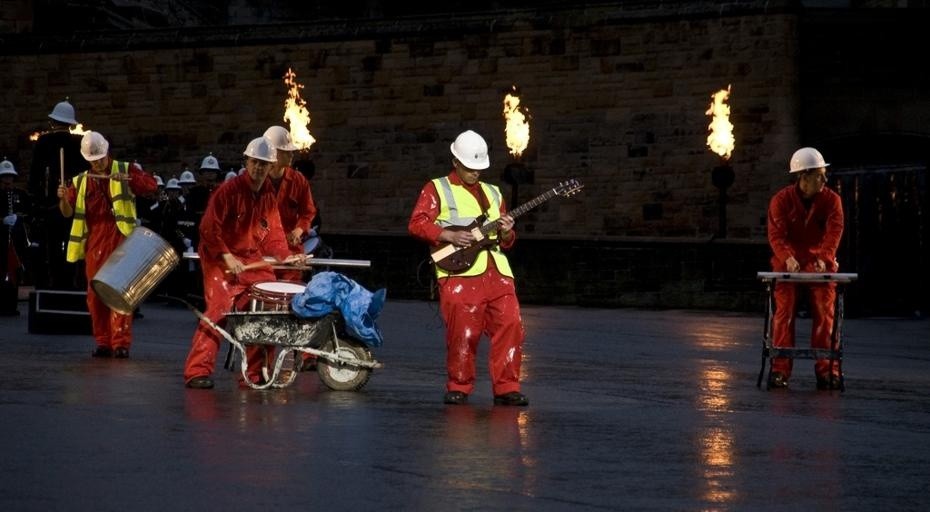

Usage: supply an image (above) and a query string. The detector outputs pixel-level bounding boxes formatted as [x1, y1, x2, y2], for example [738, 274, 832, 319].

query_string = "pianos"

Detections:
[757, 272, 859, 280]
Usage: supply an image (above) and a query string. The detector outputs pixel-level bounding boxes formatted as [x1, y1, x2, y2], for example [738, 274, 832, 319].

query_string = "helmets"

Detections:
[244, 137, 278, 162]
[225, 172, 235, 179]
[80, 132, 108, 161]
[451, 130, 489, 170]
[165, 179, 182, 189]
[177, 171, 196, 185]
[263, 126, 301, 151]
[200, 156, 221, 170]
[153, 176, 164, 186]
[0, 160, 17, 176]
[789, 147, 830, 173]
[48, 102, 77, 124]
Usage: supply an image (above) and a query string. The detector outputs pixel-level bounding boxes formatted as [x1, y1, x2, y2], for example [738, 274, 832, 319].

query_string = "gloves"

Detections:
[3, 216, 13, 226]
[11, 214, 18, 221]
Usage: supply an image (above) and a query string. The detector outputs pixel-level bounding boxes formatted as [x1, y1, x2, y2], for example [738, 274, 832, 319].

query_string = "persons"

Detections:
[185, 154, 222, 238]
[59, 132, 158, 359]
[185, 137, 309, 388]
[1, 157, 29, 317]
[29, 97, 91, 241]
[409, 130, 530, 406]
[151, 169, 197, 228]
[769, 148, 844, 391]
[264, 126, 319, 375]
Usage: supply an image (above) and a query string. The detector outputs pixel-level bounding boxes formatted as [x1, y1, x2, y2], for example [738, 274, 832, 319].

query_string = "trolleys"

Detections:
[193, 309, 385, 390]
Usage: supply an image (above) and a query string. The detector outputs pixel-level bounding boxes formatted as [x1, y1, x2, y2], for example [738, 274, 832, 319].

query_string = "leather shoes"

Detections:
[185, 377, 212, 388]
[768, 373, 789, 387]
[93, 348, 113, 356]
[494, 392, 529, 405]
[115, 348, 128, 358]
[817, 375, 845, 389]
[444, 391, 469, 403]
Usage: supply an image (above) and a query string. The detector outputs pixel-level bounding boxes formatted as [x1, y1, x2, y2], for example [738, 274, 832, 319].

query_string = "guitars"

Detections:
[430, 179, 585, 275]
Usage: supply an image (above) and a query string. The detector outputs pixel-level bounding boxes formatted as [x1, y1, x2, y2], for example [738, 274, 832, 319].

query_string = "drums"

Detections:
[250, 280, 307, 311]
[91, 226, 180, 316]
